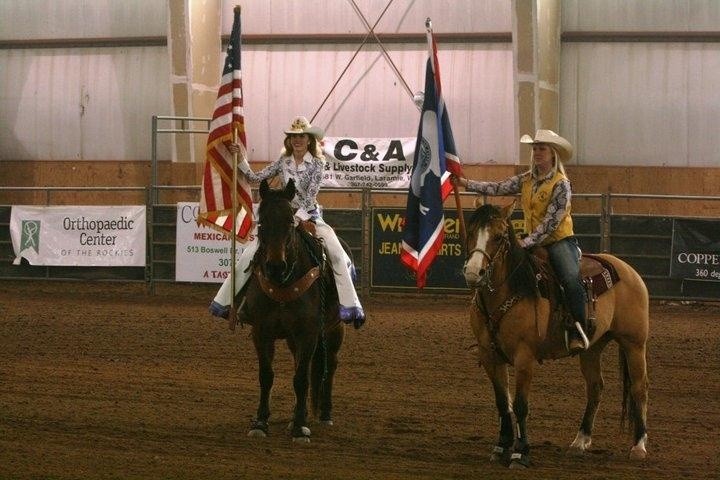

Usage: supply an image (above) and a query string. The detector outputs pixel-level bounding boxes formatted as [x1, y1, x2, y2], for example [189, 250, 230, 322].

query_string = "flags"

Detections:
[197, 6, 252, 245]
[399, 26, 461, 290]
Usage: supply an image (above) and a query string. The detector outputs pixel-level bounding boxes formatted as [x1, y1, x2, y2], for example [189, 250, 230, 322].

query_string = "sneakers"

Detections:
[570, 337, 585, 351]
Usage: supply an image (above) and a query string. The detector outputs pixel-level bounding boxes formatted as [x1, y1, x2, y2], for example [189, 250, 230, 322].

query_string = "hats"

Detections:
[519, 130, 574, 164]
[284, 116, 325, 142]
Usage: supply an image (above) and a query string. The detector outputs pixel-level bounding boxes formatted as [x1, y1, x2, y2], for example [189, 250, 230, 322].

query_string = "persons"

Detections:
[211, 115, 366, 329]
[452, 129, 588, 353]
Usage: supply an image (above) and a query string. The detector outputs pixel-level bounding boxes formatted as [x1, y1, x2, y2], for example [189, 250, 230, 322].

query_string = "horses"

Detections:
[460, 198, 652, 472]
[243, 176, 345, 444]
